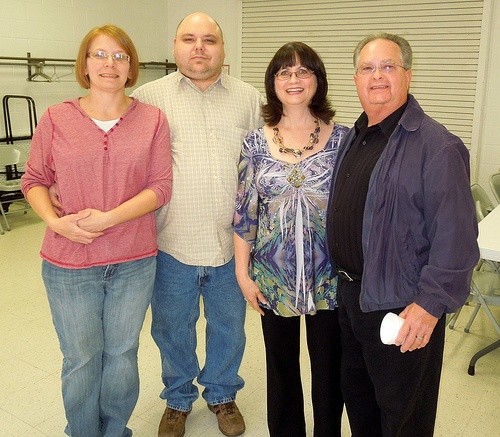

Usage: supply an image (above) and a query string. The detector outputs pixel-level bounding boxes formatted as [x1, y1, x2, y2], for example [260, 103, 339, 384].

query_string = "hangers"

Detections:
[27, 62, 84, 82]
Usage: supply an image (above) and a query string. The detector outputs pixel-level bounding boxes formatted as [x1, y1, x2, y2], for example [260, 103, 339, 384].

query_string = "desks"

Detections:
[468, 204, 500, 381]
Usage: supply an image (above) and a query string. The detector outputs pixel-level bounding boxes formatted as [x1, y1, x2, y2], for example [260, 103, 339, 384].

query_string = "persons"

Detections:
[50, 12, 268, 437]
[326, 33, 481, 437]
[20, 25, 172, 437]
[233, 41, 350, 437]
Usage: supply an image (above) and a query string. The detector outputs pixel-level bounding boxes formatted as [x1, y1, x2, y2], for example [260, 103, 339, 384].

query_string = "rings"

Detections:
[416, 335, 424, 340]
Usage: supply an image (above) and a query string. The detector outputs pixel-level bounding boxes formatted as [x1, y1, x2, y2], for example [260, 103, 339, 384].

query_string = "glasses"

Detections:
[273, 66, 315, 80]
[86, 50, 130, 64]
[356, 60, 407, 77]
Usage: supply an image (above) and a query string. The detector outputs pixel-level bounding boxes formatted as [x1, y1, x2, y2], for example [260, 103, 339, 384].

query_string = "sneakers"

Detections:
[158, 406, 191, 437]
[207, 401, 246, 437]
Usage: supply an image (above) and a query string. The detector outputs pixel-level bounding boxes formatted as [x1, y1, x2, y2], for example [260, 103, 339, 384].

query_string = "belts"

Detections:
[335, 268, 362, 282]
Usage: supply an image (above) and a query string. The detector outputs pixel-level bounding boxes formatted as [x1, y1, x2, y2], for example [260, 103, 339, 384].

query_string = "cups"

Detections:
[380, 312, 405, 345]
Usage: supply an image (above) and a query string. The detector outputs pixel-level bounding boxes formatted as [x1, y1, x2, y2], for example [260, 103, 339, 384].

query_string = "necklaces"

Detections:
[272, 115, 321, 159]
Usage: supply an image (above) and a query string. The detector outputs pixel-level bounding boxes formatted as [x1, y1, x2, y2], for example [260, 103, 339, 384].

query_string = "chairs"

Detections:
[0, 144, 28, 231]
[446, 170, 500, 332]
